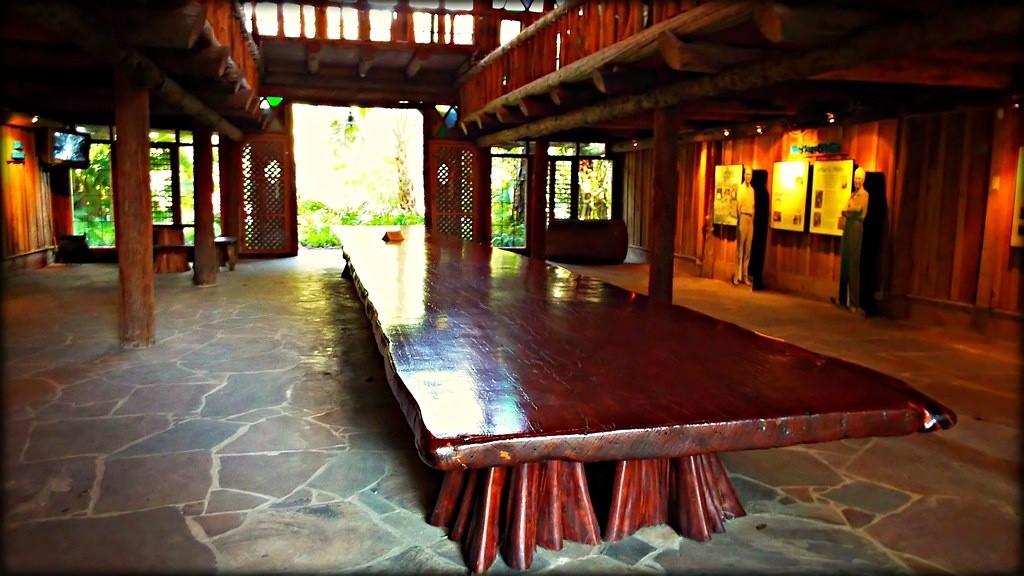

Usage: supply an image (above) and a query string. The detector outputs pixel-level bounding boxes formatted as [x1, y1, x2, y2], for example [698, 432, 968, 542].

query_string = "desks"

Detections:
[152, 223, 195, 274]
[330, 223, 926, 576]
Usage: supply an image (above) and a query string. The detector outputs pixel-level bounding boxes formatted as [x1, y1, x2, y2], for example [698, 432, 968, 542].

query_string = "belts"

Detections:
[741, 213, 752, 217]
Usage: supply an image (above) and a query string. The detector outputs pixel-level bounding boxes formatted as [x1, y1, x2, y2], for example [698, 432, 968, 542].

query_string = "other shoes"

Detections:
[733, 275, 738, 285]
[742, 274, 751, 285]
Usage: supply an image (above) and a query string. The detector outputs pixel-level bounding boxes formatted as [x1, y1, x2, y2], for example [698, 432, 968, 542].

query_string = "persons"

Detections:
[836, 166, 868, 312]
[733, 166, 755, 285]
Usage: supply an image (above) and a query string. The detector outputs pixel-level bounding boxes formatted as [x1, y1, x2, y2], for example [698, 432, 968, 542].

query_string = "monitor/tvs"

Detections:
[38, 128, 91, 167]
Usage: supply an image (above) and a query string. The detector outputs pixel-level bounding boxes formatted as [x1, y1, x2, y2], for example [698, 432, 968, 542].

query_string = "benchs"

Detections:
[155, 235, 237, 272]
